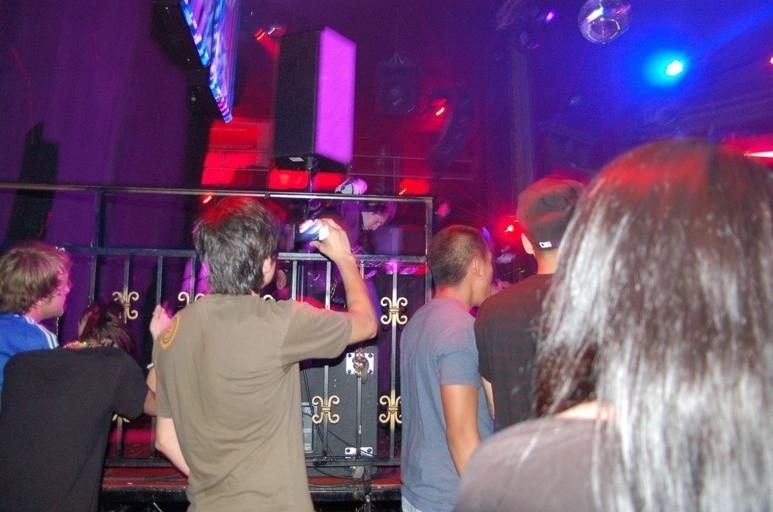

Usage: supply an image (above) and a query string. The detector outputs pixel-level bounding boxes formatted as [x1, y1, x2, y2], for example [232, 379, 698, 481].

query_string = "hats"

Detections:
[516, 180, 586, 254]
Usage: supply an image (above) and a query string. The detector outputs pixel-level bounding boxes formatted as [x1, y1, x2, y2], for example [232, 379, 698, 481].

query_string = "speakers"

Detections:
[274, 25, 356, 174]
[300, 274, 432, 474]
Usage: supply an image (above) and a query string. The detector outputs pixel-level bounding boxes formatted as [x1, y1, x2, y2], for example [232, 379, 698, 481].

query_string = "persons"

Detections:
[452, 136, 771, 511]
[470, 172, 587, 435]
[151, 198, 379, 511]
[0, 302, 172, 510]
[300, 174, 398, 438]
[0, 241, 71, 391]
[397, 223, 498, 512]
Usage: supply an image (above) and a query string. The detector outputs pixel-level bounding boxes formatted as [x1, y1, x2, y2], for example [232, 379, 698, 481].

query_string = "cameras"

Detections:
[295, 215, 330, 244]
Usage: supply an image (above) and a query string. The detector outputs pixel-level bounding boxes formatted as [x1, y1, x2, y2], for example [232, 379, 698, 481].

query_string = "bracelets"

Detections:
[146, 362, 154, 370]
